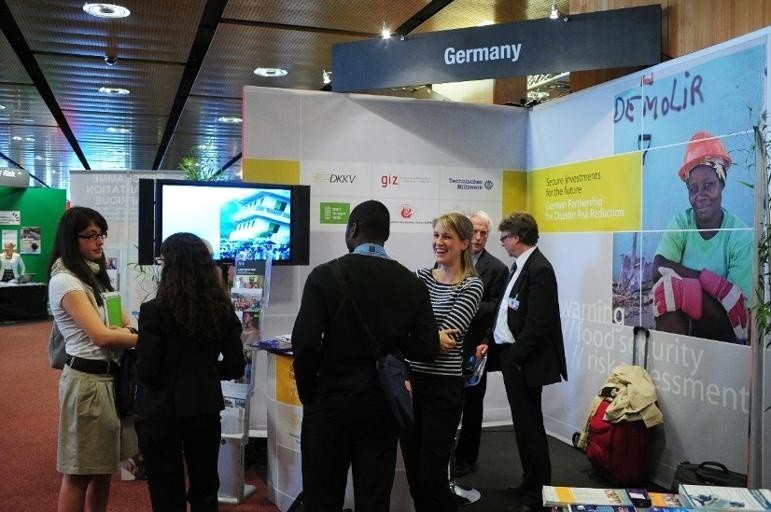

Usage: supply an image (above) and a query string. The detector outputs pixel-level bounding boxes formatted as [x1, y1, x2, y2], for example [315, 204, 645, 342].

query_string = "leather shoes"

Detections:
[451, 459, 480, 478]
[506, 481, 552, 512]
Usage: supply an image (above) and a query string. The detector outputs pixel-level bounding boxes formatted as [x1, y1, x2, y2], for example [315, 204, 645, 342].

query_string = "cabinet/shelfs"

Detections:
[0, 280, 48, 324]
[217, 250, 273, 505]
[264, 349, 410, 511]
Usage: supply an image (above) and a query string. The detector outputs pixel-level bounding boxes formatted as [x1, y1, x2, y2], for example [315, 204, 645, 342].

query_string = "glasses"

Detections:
[500, 233, 511, 242]
[78, 231, 107, 241]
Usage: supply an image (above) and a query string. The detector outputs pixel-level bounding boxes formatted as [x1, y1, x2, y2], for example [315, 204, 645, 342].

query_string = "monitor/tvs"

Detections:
[136, 177, 310, 267]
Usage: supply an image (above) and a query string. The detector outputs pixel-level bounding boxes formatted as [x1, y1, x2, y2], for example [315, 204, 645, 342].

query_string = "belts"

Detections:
[64, 354, 117, 375]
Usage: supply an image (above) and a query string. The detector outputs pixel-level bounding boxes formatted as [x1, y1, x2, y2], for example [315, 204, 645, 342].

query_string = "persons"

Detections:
[398, 213, 485, 512]
[134, 232, 247, 512]
[291, 199, 440, 512]
[0, 241, 26, 282]
[650, 131, 754, 345]
[475, 212, 568, 512]
[450, 210, 510, 477]
[47, 207, 139, 512]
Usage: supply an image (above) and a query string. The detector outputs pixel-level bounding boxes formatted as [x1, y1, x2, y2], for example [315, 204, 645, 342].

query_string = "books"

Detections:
[214, 260, 266, 384]
[541, 483, 771, 512]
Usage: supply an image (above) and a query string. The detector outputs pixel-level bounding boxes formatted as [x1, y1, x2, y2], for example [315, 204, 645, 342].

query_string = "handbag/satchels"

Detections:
[376, 354, 414, 433]
[47, 319, 66, 370]
[114, 349, 138, 420]
[670, 461, 747, 493]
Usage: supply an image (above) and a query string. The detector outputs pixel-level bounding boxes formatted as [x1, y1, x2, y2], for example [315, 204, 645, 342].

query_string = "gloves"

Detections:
[648, 266, 704, 320]
[699, 268, 750, 339]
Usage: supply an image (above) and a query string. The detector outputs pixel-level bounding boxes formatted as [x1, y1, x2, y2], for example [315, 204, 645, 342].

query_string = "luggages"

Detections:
[586, 326, 650, 483]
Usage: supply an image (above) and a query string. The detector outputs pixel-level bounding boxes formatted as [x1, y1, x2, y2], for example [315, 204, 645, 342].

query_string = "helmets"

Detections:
[678, 131, 732, 183]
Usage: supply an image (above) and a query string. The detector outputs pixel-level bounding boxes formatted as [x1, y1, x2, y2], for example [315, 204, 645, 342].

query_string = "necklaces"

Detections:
[432, 269, 460, 305]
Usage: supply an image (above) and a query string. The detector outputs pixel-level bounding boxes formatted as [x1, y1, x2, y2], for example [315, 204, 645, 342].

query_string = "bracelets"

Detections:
[125, 326, 138, 334]
